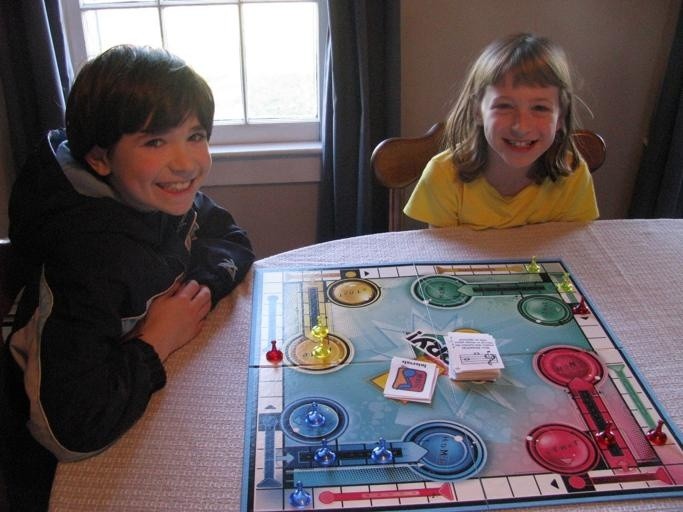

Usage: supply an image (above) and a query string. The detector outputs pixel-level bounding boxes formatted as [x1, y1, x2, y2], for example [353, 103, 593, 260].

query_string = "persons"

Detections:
[402, 32, 604, 231]
[0, 44, 258, 511]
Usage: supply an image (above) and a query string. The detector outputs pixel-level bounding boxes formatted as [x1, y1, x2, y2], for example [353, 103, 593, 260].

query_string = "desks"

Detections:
[46, 218, 681, 512]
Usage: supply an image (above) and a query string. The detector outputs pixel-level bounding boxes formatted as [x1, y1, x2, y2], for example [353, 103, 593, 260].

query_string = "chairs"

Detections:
[366, 117, 611, 234]
[0, 236, 26, 347]
